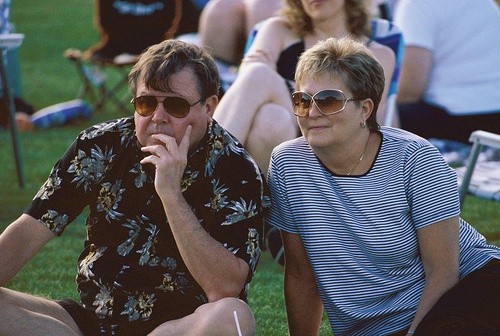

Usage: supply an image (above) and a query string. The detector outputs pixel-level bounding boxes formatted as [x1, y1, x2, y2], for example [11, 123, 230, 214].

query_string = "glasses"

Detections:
[130, 95, 201, 118]
[291, 88, 359, 116]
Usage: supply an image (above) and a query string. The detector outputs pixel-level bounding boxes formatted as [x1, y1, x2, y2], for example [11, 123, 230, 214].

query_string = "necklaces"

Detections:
[345, 128, 372, 177]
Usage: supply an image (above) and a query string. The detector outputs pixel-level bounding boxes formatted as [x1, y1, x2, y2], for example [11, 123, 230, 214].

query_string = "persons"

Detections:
[260, 37, 499, 335]
[378, 0, 500, 145]
[196, 0, 289, 96]
[211, 0, 396, 195]
[1, 37, 271, 336]
[84, 0, 199, 68]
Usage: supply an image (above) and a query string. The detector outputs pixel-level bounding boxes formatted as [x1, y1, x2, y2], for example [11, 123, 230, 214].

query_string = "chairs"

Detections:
[64, 1, 184, 117]
[460, 131, 500, 213]
[244, 15, 404, 126]
